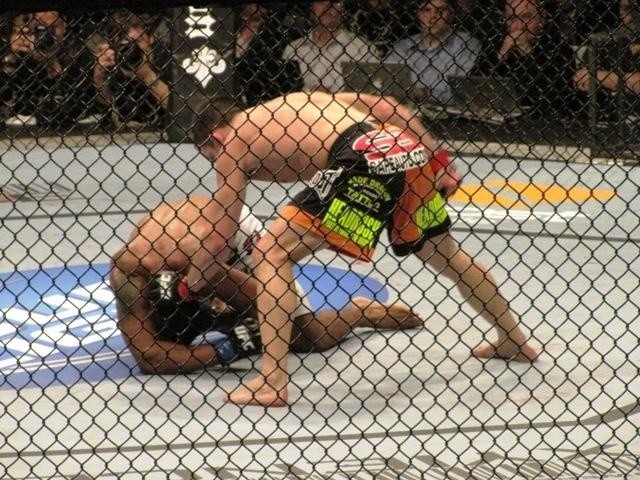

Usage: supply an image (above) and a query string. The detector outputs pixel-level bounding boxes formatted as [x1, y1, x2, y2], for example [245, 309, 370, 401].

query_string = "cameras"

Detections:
[115, 40, 144, 70]
[33, 25, 56, 51]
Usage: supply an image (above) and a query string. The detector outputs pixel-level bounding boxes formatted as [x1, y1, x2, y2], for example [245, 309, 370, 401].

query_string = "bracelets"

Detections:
[426, 148, 452, 179]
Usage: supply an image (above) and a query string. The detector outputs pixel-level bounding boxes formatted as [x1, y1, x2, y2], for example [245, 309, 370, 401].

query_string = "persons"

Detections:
[146, 86, 539, 408]
[1, 0, 639, 127]
[109, 192, 424, 377]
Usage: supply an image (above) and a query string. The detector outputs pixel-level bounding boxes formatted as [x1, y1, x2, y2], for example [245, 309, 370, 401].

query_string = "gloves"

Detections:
[149, 272, 194, 310]
[213, 317, 263, 365]
[429, 149, 461, 198]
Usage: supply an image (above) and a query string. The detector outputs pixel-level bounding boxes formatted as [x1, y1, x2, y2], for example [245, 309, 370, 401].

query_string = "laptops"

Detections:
[447, 75, 514, 110]
[235, 57, 306, 106]
[340, 63, 412, 104]
